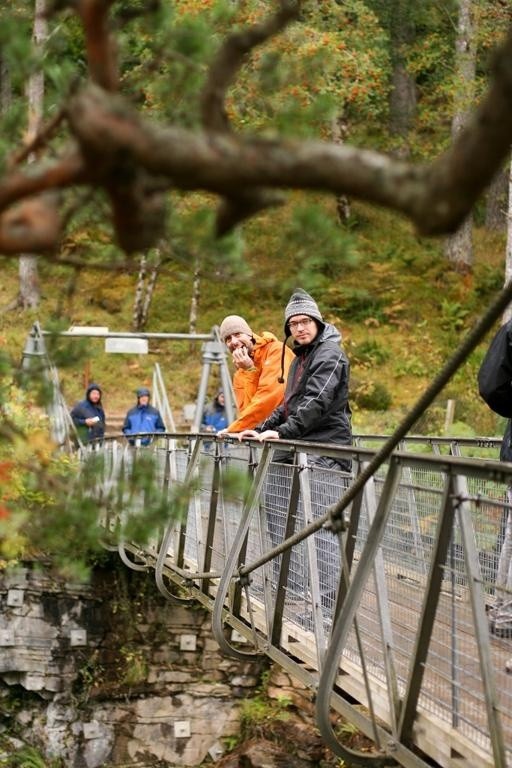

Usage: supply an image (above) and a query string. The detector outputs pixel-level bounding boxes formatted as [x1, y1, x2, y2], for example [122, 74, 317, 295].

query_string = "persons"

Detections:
[123, 388, 166, 446]
[69, 385, 105, 450]
[478, 312, 512, 638]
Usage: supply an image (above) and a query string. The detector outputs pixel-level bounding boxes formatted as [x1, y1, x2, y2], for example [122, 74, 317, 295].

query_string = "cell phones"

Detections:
[92, 416, 100, 422]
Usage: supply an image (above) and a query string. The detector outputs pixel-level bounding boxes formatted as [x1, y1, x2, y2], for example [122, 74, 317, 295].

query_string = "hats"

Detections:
[282, 288, 325, 338]
[219, 314, 253, 344]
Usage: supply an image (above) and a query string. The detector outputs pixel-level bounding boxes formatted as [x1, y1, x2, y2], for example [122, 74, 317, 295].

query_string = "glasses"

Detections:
[286, 317, 314, 329]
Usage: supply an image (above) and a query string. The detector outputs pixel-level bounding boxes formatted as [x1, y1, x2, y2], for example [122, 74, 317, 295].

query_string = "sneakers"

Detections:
[486, 619, 512, 638]
[252, 574, 333, 634]
